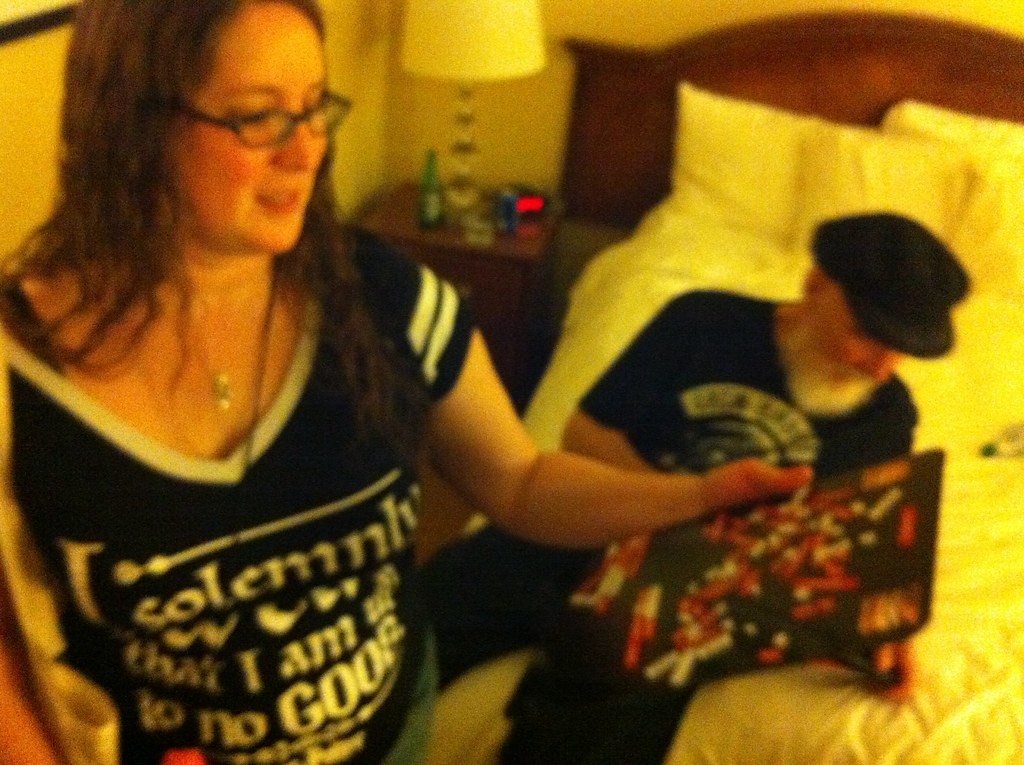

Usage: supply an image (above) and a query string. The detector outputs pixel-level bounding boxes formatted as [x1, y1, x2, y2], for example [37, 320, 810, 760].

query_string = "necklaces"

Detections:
[209, 366, 238, 416]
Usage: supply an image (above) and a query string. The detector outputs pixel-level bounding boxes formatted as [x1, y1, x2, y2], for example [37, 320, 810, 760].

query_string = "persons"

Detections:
[0, 0, 811, 764]
[415, 213, 968, 765]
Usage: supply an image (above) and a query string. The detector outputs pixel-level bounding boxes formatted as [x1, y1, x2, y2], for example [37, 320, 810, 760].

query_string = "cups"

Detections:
[462, 201, 496, 246]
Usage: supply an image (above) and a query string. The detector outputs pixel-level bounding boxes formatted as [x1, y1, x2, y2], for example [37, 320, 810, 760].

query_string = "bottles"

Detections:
[421, 150, 442, 230]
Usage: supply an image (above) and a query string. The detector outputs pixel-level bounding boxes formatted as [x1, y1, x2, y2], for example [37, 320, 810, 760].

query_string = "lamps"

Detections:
[398, 0, 548, 206]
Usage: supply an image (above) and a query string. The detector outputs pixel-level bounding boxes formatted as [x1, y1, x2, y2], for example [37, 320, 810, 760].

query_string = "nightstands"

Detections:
[353, 182, 565, 394]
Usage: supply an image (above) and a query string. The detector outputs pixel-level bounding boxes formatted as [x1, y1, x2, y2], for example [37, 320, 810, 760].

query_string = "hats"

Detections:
[810, 212, 973, 359]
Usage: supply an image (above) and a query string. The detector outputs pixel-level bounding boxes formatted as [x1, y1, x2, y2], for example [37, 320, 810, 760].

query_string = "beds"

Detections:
[414, 12, 1024, 765]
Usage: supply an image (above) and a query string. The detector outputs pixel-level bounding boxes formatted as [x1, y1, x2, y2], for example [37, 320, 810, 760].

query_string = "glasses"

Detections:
[171, 92, 353, 150]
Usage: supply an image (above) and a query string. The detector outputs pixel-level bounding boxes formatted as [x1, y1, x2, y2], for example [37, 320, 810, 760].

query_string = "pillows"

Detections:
[671, 83, 967, 253]
[876, 101, 1024, 303]
